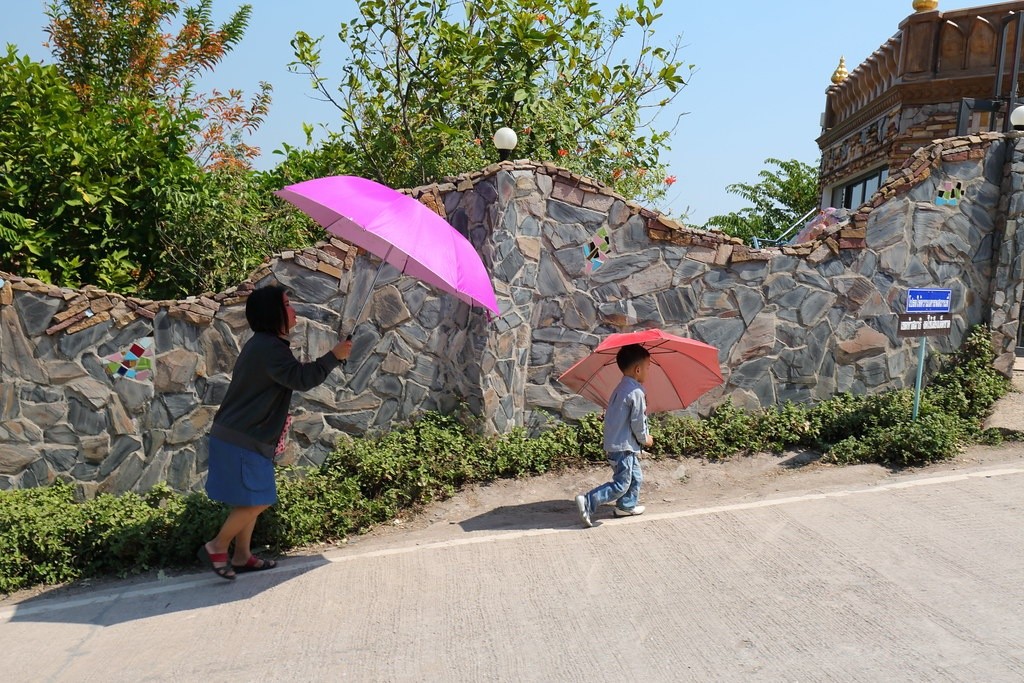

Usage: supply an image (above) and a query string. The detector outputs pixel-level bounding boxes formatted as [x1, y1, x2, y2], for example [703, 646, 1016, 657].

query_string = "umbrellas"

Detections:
[274, 176, 500, 366]
[556, 328, 725, 450]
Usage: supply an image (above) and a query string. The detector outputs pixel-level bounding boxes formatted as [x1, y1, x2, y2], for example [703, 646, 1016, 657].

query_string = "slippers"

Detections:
[197, 546, 237, 579]
[231, 556, 276, 573]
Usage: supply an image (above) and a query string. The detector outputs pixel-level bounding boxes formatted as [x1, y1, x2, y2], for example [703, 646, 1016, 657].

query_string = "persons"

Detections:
[204, 286, 353, 580]
[575, 344, 654, 527]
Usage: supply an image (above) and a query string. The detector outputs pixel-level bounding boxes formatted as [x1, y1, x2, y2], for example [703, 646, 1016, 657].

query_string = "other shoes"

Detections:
[613, 506, 645, 517]
[575, 495, 593, 526]
